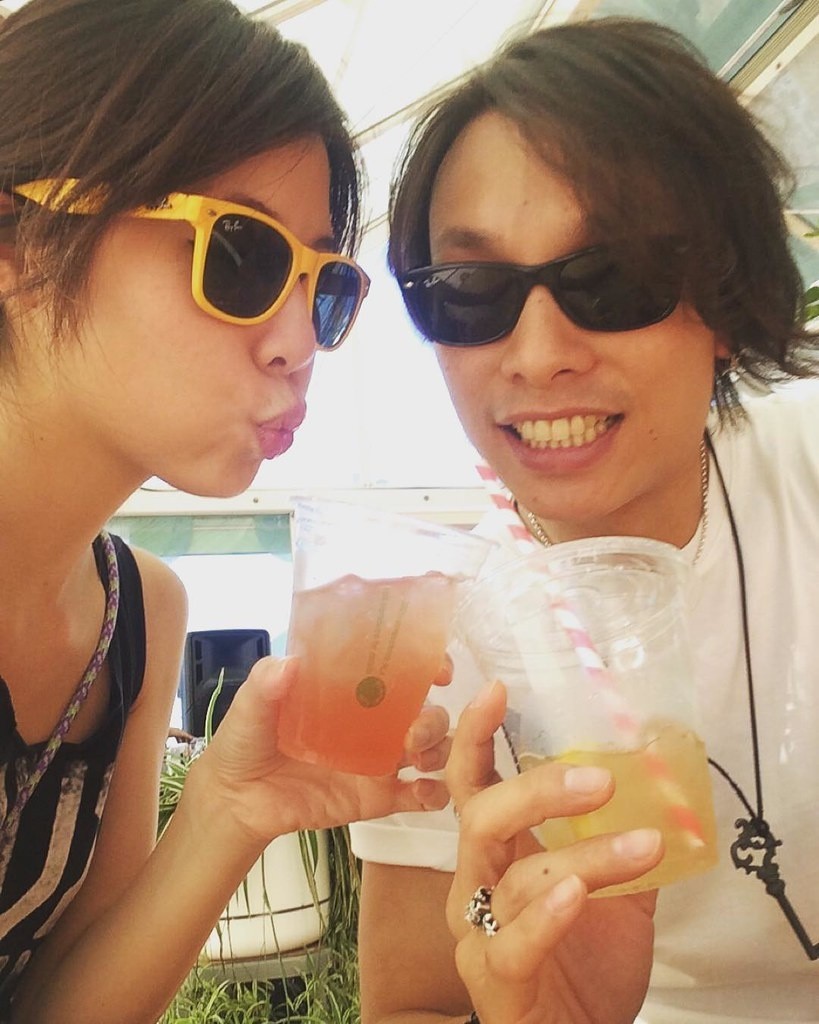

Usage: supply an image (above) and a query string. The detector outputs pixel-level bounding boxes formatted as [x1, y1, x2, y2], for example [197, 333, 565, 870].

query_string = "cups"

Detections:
[279, 492, 494, 778]
[451, 534, 720, 898]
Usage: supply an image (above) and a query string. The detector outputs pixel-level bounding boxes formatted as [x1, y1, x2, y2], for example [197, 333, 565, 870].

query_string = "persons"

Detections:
[0, 2, 453, 1024]
[356, 21, 819, 1021]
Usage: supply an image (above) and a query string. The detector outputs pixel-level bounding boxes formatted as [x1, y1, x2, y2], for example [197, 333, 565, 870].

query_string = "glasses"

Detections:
[11, 178, 371, 351]
[393, 232, 689, 348]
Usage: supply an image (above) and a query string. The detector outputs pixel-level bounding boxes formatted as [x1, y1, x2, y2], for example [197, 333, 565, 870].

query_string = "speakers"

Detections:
[176, 628, 271, 744]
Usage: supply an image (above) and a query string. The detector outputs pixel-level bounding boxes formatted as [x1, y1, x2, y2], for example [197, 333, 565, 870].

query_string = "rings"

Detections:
[466, 886, 501, 936]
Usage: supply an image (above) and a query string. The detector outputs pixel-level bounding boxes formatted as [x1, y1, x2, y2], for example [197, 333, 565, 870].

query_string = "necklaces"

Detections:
[527, 434, 711, 582]
[512, 425, 819, 963]
